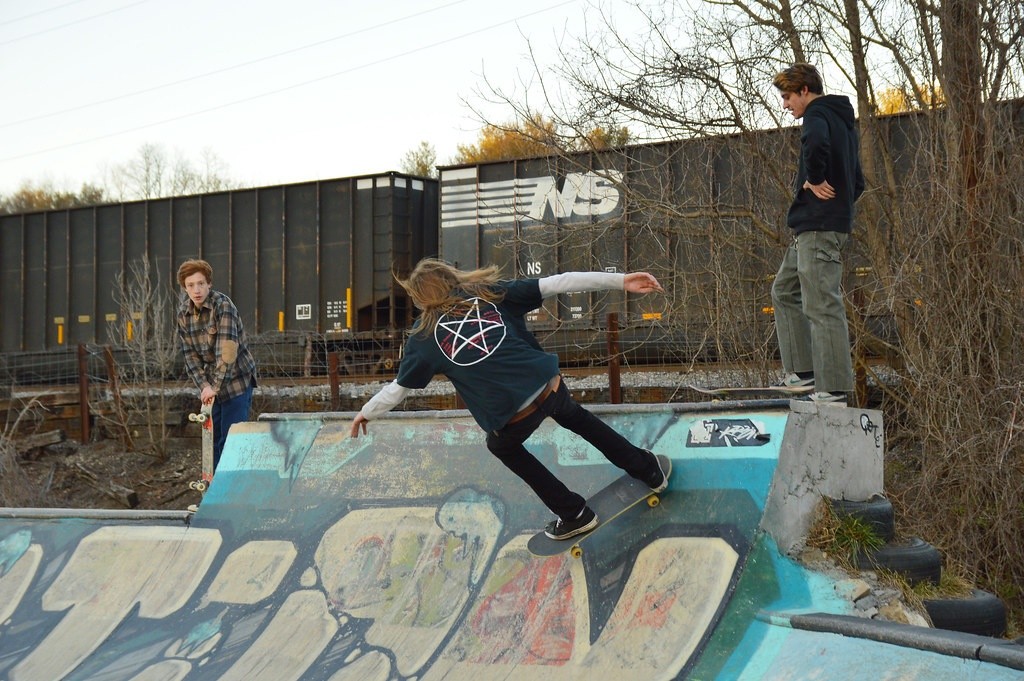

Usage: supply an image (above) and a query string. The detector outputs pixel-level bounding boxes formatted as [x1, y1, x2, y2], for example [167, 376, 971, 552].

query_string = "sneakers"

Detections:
[794, 389, 848, 406]
[544, 505, 599, 540]
[640, 447, 668, 493]
[770, 371, 815, 388]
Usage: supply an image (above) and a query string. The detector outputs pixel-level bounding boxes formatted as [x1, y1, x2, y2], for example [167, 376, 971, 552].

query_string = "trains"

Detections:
[1, 94, 1024, 386]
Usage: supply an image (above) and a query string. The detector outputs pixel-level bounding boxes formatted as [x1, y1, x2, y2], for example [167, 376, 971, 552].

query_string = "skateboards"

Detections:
[527, 453, 672, 558]
[188, 396, 215, 500]
[687, 383, 814, 401]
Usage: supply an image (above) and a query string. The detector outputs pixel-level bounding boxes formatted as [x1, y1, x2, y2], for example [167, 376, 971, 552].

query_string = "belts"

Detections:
[508, 384, 552, 424]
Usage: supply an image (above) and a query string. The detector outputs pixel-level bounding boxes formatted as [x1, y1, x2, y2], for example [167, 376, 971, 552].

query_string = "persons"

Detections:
[771, 63, 866, 406]
[349, 258, 668, 542]
[177, 259, 259, 476]
